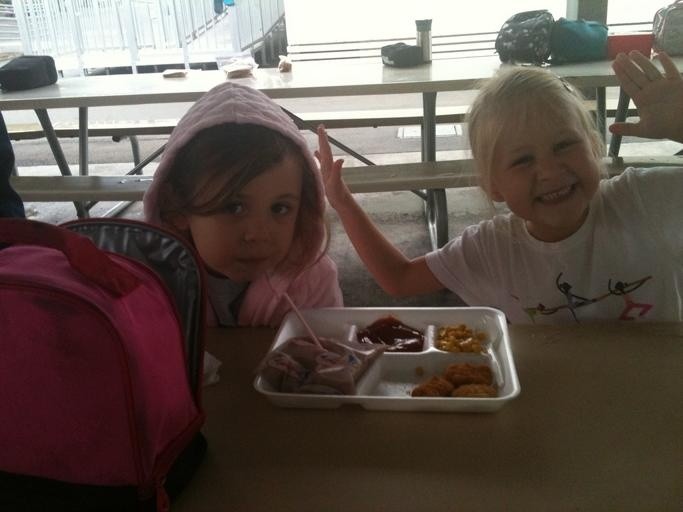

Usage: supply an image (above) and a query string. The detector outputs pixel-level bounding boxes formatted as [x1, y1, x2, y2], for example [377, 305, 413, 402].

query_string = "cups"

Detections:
[415, 19, 432, 64]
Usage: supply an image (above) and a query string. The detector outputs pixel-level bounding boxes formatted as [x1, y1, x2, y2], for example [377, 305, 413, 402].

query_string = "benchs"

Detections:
[6, 158, 683, 293]
[1, 92, 636, 176]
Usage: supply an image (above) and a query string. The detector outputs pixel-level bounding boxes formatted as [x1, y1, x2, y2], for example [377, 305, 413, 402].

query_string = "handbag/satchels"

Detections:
[652, 0, 683, 56]
[550, 17, 608, 65]
[1, 217, 205, 492]
[0, 56, 58, 91]
[495, 9, 554, 64]
[381, 43, 422, 68]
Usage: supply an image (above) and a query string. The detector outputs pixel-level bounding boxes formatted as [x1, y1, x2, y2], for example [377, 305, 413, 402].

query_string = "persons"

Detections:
[0, 112, 26, 221]
[309, 51, 683, 328]
[142, 83, 341, 334]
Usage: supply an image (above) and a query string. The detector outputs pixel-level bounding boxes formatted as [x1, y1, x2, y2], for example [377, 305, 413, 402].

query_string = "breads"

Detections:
[412, 369, 451, 398]
[441, 363, 492, 383]
[451, 382, 495, 401]
